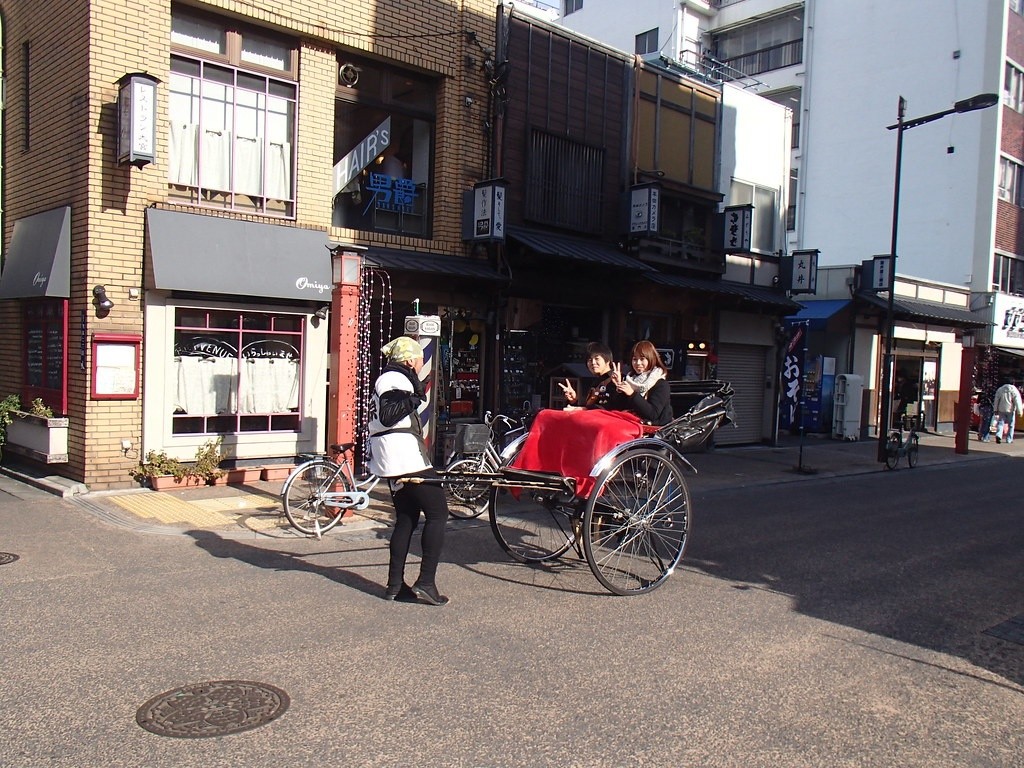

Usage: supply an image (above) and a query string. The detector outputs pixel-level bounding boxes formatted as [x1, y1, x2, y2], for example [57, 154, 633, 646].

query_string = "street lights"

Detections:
[876, 93, 999, 462]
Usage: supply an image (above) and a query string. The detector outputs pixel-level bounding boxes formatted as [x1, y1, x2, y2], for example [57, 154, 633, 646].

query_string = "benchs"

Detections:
[641, 392, 711, 433]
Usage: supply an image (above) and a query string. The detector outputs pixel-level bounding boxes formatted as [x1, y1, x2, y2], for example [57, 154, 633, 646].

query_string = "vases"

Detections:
[226, 465, 265, 483]
[262, 465, 310, 480]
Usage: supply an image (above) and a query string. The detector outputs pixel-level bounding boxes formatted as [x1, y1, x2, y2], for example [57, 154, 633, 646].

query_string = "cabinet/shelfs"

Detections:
[371, 175, 427, 237]
[550, 376, 596, 410]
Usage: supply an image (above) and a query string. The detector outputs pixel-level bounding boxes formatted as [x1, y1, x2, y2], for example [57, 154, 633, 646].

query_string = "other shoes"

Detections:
[983, 440, 990, 442]
[385, 583, 417, 600]
[996, 436, 1001, 443]
[411, 583, 448, 606]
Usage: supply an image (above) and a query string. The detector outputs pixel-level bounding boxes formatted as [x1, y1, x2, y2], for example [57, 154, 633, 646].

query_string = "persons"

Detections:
[612, 342, 673, 471]
[994, 377, 1022, 444]
[976, 382, 996, 442]
[558, 342, 621, 410]
[895, 375, 914, 417]
[365, 337, 450, 607]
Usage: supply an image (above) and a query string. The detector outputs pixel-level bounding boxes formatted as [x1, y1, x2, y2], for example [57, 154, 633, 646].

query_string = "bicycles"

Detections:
[437, 408, 545, 520]
[883, 409, 923, 470]
[279, 440, 386, 542]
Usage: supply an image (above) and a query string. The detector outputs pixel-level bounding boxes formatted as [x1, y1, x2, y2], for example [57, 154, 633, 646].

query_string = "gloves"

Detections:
[409, 367, 433, 396]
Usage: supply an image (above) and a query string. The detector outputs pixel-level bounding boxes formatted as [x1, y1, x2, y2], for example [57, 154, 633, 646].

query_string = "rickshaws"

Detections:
[394, 379, 736, 596]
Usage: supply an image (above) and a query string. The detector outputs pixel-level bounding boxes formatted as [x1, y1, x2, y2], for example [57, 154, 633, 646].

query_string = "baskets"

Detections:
[455, 423, 490, 454]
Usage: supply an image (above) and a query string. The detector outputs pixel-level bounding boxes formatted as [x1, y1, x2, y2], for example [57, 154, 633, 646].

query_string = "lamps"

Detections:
[962, 330, 974, 349]
[93, 284, 115, 310]
[314, 302, 330, 320]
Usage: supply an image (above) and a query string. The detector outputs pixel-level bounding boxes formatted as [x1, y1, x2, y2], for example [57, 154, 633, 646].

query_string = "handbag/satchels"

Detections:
[991, 413, 999, 427]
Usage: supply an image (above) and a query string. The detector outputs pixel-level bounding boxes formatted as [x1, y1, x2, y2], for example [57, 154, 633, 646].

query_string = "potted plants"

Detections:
[128, 435, 229, 490]
[0, 393, 69, 464]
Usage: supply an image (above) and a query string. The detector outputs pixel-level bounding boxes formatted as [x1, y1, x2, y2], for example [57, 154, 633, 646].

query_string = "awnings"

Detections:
[855, 291, 996, 333]
[359, 228, 852, 335]
[998, 348, 1024, 359]
